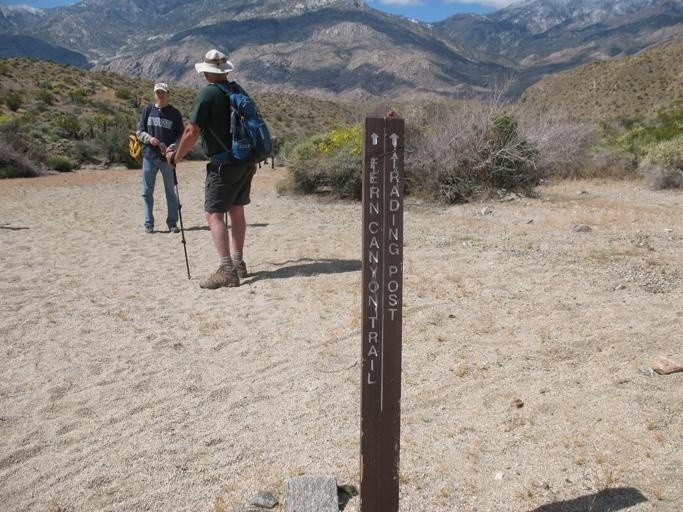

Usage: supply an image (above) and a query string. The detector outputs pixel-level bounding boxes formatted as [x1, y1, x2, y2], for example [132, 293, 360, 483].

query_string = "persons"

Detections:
[165, 48, 256, 290]
[136, 82, 184, 233]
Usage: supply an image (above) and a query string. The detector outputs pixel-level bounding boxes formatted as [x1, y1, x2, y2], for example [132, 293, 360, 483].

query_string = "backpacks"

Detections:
[204, 81, 272, 164]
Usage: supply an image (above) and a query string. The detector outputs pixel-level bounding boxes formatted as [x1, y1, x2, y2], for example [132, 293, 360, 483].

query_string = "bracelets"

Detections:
[169, 151, 177, 164]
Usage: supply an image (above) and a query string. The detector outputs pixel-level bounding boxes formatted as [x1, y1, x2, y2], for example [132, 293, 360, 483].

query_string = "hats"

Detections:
[195, 49, 234, 73]
[154, 83, 169, 93]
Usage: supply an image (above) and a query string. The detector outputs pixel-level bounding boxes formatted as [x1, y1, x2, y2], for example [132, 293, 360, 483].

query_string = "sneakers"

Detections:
[169, 225, 180, 233]
[199, 261, 246, 288]
[145, 226, 153, 232]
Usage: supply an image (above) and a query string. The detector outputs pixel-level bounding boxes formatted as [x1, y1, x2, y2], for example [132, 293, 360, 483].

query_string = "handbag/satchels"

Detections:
[129, 133, 143, 163]
